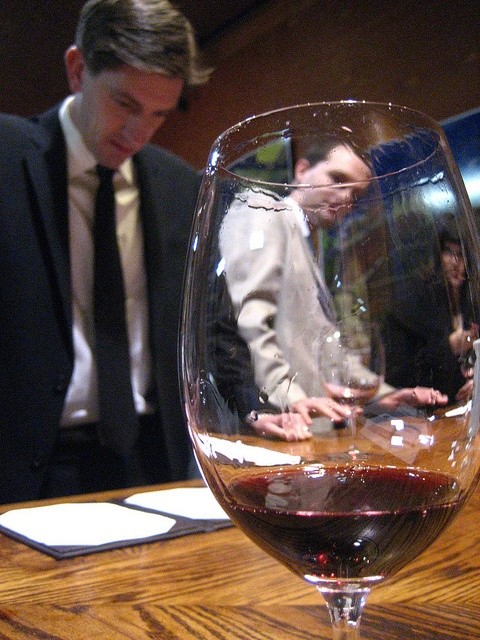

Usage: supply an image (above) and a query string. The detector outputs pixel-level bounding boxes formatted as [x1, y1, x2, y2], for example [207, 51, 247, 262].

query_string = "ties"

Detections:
[92, 164, 140, 456]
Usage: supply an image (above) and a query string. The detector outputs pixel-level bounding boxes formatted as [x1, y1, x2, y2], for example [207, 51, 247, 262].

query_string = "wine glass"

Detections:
[318, 322, 387, 462]
[176, 100, 479, 639]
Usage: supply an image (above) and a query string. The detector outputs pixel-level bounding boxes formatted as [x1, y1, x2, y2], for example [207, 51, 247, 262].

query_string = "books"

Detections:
[1, 486, 233, 562]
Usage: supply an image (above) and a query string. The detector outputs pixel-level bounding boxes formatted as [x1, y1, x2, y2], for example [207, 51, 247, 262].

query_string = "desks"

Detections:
[0, 467, 479, 639]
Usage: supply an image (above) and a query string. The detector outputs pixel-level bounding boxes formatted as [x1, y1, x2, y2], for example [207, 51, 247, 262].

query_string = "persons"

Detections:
[387, 225, 478, 406]
[215, 124, 445, 429]
[0, 3, 313, 508]
